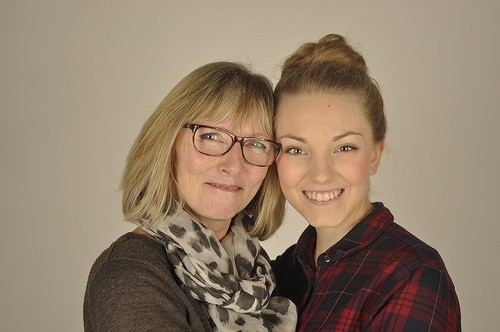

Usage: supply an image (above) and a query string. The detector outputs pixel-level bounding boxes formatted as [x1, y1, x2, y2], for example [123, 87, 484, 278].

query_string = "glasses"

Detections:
[182, 124, 282, 167]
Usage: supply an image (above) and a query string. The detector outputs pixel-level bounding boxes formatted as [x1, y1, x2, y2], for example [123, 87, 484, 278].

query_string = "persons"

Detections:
[267, 32, 462, 332]
[82, 61, 298, 332]
